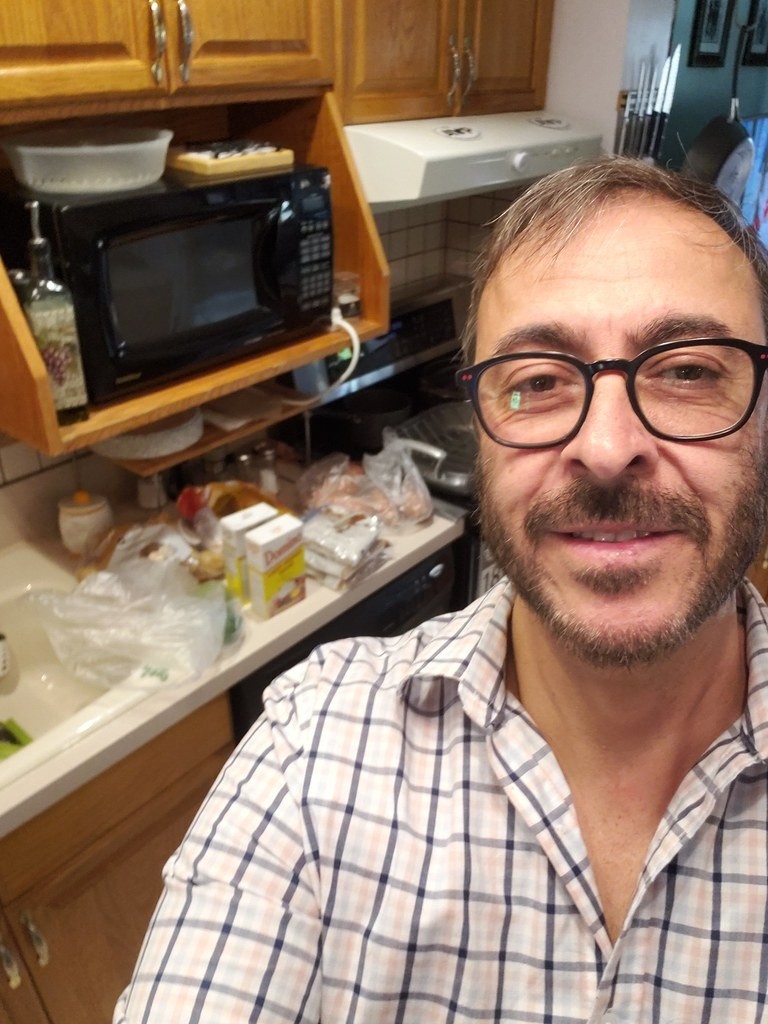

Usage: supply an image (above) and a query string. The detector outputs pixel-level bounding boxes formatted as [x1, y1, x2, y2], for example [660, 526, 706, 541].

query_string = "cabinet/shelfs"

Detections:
[0, 685, 234, 1024]
[0, 0, 557, 454]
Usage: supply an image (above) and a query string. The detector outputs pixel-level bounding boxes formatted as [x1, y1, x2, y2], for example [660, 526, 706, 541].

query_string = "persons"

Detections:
[112, 155, 767, 1024]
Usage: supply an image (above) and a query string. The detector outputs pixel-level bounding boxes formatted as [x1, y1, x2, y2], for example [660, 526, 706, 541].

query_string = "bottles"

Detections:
[234, 445, 280, 498]
[21, 236, 90, 427]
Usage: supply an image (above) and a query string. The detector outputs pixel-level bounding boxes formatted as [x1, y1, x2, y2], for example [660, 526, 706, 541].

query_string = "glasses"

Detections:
[455, 337, 768, 449]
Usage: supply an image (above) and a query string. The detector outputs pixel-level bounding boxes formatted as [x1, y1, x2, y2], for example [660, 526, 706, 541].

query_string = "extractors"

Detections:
[342, 109, 603, 214]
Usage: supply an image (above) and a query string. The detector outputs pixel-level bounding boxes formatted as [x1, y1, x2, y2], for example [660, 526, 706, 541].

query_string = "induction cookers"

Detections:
[278, 276, 490, 507]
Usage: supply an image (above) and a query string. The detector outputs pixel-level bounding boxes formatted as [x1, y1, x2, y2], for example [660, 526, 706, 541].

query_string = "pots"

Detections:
[679, 26, 755, 208]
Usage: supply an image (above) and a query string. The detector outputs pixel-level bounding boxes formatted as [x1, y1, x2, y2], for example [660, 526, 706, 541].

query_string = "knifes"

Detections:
[629, 43, 682, 159]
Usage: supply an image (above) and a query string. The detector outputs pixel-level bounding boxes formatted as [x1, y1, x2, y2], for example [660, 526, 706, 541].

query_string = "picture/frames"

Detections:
[740, 0, 768, 67]
[687, 0, 735, 68]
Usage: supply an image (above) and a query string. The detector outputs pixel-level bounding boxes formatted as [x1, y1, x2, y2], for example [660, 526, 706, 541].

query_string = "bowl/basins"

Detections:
[2, 126, 180, 198]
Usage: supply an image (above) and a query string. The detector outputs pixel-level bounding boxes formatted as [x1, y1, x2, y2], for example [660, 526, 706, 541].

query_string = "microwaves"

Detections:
[12, 164, 331, 405]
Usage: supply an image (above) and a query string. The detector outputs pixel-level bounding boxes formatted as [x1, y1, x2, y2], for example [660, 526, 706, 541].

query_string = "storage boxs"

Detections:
[219, 500, 308, 621]
